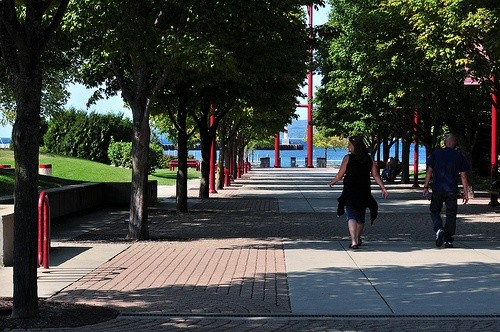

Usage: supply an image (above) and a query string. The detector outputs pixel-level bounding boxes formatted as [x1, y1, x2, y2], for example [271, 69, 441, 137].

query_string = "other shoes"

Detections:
[358, 239, 362, 246]
[435, 227, 445, 247]
[349, 244, 358, 249]
[445, 242, 453, 248]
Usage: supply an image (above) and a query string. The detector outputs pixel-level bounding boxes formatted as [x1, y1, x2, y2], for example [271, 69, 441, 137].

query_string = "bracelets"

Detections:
[424, 186, 429, 188]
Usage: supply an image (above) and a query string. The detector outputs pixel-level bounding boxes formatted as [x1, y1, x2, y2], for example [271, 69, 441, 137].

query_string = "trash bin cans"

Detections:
[291, 157, 296, 168]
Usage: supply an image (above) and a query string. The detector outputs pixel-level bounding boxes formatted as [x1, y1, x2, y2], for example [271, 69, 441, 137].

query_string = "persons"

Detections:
[329, 136, 388, 249]
[423, 134, 469, 246]
